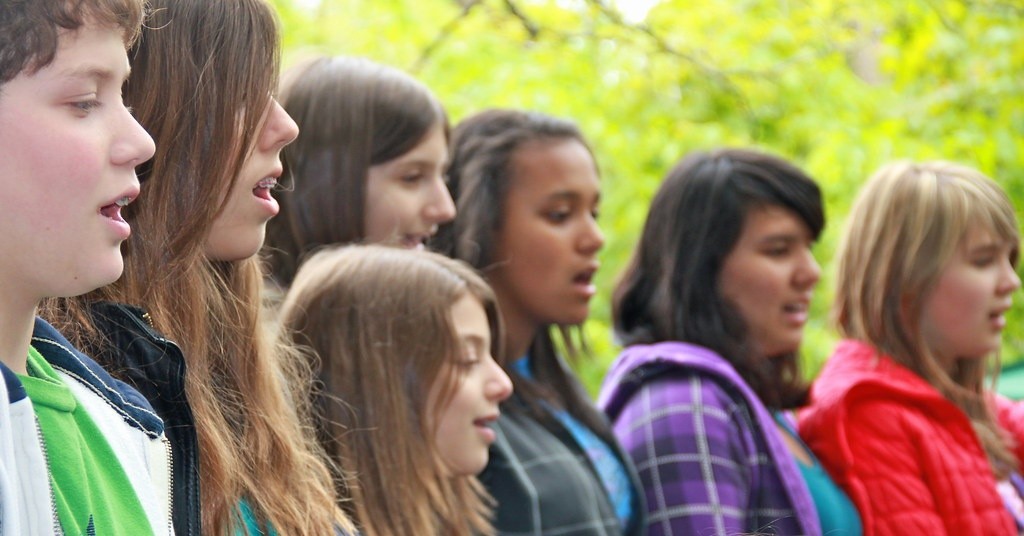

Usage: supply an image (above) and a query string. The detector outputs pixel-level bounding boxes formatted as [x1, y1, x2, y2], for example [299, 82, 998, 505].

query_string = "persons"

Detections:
[417, 103, 650, 536]
[253, 49, 462, 356]
[0, 2, 204, 536]
[786, 157, 1024, 536]
[269, 240, 518, 536]
[593, 144, 866, 536]
[33, 0, 364, 536]
